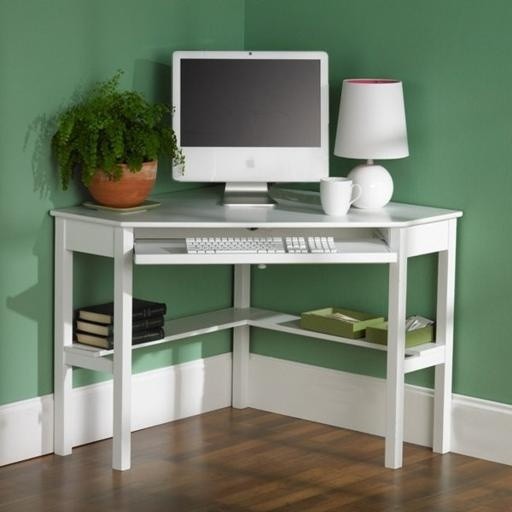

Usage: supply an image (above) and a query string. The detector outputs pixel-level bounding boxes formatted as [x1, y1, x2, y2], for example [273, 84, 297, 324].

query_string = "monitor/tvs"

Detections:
[169, 50, 330, 206]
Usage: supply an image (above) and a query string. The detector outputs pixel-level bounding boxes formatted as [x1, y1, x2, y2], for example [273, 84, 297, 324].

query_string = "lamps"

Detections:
[332, 79, 410, 208]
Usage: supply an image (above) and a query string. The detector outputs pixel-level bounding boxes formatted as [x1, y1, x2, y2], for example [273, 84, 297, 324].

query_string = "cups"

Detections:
[320, 176, 362, 218]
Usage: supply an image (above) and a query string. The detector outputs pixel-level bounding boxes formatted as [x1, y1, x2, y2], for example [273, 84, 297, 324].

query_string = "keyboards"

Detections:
[184, 234, 337, 254]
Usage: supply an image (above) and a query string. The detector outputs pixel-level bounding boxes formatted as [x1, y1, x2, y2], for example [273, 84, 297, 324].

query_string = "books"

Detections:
[73, 327, 164, 350]
[77, 297, 167, 324]
[70, 315, 164, 337]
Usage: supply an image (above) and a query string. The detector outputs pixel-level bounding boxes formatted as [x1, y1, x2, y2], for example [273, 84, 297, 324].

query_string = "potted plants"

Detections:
[43, 67, 186, 206]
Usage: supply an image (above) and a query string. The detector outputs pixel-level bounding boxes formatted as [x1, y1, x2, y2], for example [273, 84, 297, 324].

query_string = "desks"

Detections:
[47, 186, 463, 472]
